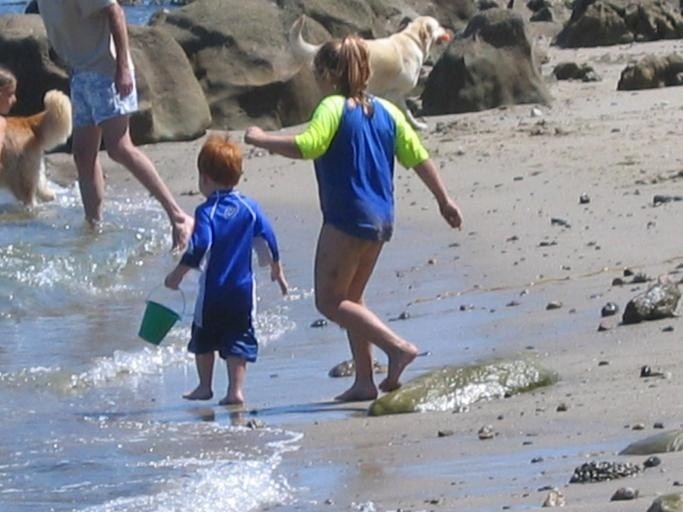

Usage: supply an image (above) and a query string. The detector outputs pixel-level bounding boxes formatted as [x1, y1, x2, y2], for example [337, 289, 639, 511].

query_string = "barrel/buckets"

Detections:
[137, 284, 186, 346]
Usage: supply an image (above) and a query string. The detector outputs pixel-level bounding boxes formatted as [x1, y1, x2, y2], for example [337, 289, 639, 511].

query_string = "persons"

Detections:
[0, 66, 17, 156]
[243, 34, 465, 403]
[163, 131, 289, 406]
[34, 0, 197, 257]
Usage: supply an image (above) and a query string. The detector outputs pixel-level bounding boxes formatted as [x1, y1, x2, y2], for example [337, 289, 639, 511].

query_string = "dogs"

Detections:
[288, 15, 448, 131]
[0, 90, 73, 212]
